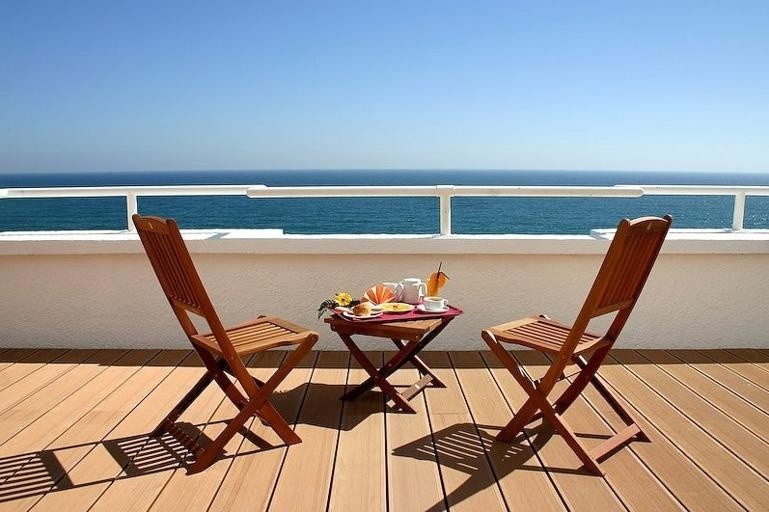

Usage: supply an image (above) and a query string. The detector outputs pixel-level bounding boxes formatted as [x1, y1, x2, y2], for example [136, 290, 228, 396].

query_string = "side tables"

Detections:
[324, 312, 455, 414]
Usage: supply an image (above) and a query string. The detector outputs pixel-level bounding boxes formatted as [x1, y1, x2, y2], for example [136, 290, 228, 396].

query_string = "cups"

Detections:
[383, 282, 405, 303]
[427, 279, 440, 297]
[403, 279, 428, 303]
[424, 297, 449, 310]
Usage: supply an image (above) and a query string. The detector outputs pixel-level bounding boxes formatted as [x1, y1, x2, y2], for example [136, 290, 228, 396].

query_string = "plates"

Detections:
[342, 308, 385, 319]
[375, 303, 416, 313]
[326, 298, 465, 324]
[418, 306, 450, 314]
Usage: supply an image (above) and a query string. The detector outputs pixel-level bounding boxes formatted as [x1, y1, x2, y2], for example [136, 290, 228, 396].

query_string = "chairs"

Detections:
[481, 215, 672, 477]
[131, 214, 319, 475]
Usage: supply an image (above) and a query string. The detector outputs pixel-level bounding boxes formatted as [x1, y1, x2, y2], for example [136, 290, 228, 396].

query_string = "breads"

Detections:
[353, 305, 371, 315]
